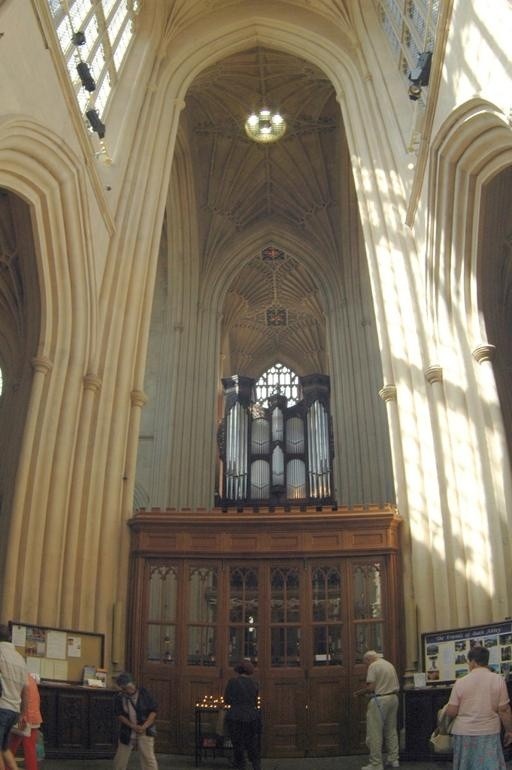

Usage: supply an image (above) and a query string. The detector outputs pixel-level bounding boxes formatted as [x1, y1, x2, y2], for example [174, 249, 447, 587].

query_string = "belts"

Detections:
[375, 692, 395, 697]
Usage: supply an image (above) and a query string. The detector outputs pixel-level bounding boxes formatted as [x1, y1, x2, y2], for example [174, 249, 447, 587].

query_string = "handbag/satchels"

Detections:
[8, 722, 32, 737]
[146, 726, 158, 737]
[35, 730, 46, 761]
[255, 711, 263, 734]
[215, 704, 230, 737]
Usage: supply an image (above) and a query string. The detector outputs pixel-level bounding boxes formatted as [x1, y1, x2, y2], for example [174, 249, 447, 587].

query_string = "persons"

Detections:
[222, 656, 262, 770]
[7, 643, 44, 770]
[112, 671, 163, 769]
[0, 622, 32, 769]
[351, 649, 403, 770]
[427, 646, 510, 769]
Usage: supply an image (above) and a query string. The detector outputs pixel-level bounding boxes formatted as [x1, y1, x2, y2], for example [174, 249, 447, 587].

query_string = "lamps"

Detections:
[70, 28, 105, 139]
[406, 51, 433, 101]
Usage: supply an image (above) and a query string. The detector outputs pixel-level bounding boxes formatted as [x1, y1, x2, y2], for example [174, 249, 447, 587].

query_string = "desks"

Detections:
[404, 683, 457, 762]
[38, 682, 119, 760]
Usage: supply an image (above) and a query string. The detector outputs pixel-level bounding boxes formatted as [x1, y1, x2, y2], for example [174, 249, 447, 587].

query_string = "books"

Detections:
[82, 664, 108, 689]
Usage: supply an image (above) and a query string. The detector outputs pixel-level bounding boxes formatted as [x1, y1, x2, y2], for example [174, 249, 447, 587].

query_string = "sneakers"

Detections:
[384, 760, 400, 767]
[361, 762, 384, 769]
